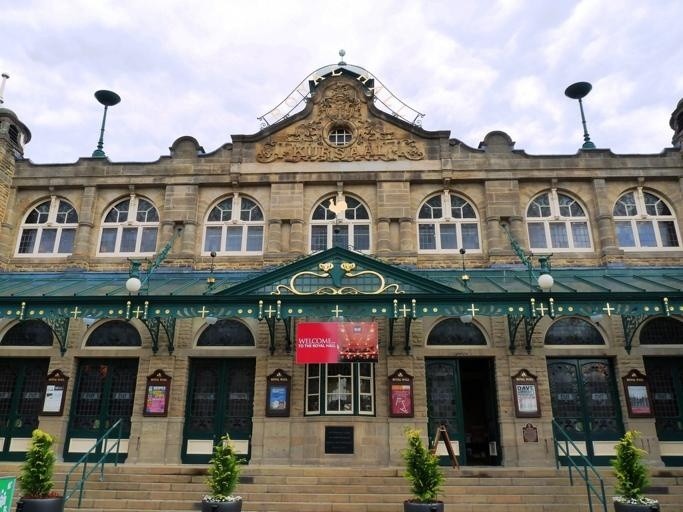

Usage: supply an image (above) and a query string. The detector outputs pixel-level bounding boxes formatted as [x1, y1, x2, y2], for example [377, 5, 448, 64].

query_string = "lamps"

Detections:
[206, 250, 217, 287]
[89, 88, 122, 159]
[564, 79, 597, 149]
[459, 246, 470, 286]
[125, 258, 143, 294]
[537, 253, 554, 292]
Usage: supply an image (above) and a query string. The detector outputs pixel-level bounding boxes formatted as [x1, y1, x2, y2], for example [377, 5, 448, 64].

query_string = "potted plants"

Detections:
[198, 428, 248, 512]
[400, 423, 445, 512]
[16, 426, 65, 512]
[609, 427, 660, 512]
